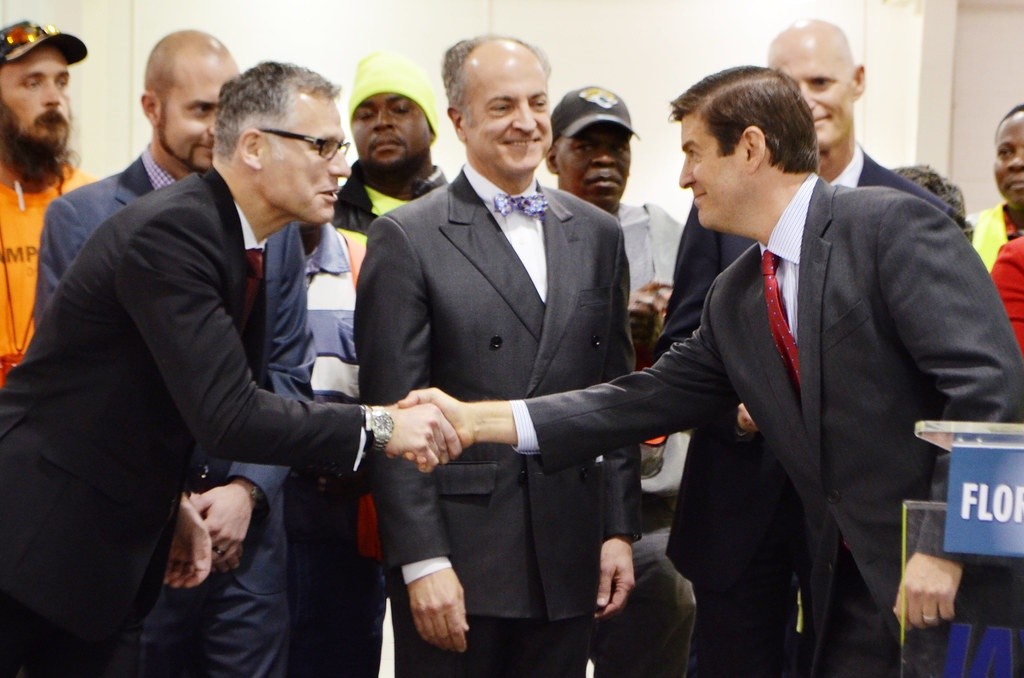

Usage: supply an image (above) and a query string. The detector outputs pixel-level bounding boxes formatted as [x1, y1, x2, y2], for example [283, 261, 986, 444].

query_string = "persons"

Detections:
[285, 216, 360, 676]
[330, 53, 451, 678]
[890, 100, 1024, 353]
[534, 86, 702, 678]
[0, 22, 98, 388]
[0, 64, 464, 678]
[352, 33, 648, 678]
[646, 17, 969, 678]
[380, 61, 1024, 677]
[35, 29, 317, 678]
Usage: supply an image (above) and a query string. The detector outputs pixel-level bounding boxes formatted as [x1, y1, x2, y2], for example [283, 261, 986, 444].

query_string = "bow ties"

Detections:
[494, 193, 548, 221]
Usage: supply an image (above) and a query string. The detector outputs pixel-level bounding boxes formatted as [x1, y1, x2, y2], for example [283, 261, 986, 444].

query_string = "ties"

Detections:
[241, 248, 263, 331]
[761, 249, 801, 392]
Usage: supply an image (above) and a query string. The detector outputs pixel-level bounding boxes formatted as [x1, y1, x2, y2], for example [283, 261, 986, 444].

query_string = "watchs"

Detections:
[247, 484, 264, 502]
[368, 405, 396, 453]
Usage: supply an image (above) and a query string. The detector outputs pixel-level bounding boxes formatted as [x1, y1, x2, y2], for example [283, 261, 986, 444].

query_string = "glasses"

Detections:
[257, 127, 350, 160]
[0, 24, 62, 46]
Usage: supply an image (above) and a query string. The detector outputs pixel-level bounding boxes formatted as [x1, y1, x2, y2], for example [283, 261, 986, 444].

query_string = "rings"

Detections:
[922, 615, 939, 623]
[213, 544, 226, 558]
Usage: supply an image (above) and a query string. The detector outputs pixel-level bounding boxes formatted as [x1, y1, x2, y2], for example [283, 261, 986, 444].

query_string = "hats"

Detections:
[550, 86, 640, 142]
[349, 50, 438, 148]
[0, 21, 87, 65]
